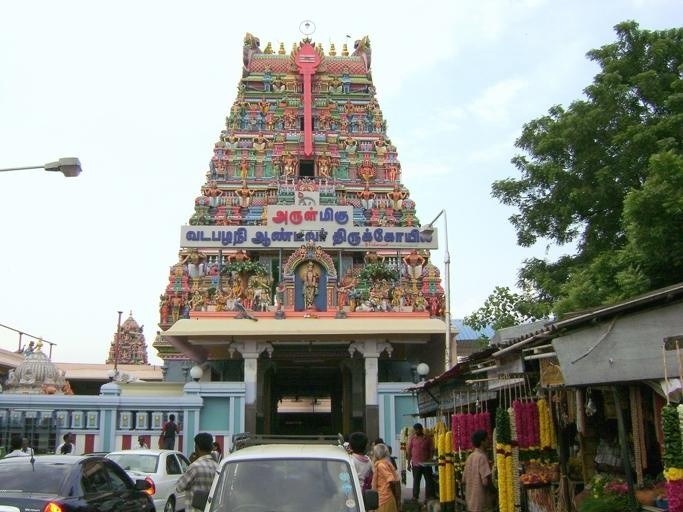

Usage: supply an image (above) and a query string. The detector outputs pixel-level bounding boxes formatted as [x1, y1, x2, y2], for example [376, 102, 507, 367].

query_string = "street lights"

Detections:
[190, 366, 204, 383]
[416, 363, 431, 381]
[106, 369, 117, 383]
[420, 207, 453, 373]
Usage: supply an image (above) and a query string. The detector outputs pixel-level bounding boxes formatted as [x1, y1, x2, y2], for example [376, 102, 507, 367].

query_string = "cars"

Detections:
[0, 450, 155, 511]
[104, 448, 194, 512]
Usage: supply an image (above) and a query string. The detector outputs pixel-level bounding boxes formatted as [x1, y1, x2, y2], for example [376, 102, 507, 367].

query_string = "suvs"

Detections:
[203, 431, 382, 512]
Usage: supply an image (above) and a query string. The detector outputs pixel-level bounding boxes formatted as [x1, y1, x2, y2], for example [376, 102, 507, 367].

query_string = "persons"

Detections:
[354, 184, 376, 211]
[385, 185, 407, 209]
[223, 131, 240, 149]
[406, 422, 439, 501]
[254, 130, 268, 151]
[314, 80, 384, 133]
[358, 160, 375, 182]
[209, 441, 222, 463]
[201, 181, 226, 209]
[372, 135, 388, 156]
[237, 160, 250, 180]
[347, 431, 374, 501]
[301, 262, 317, 308]
[364, 442, 402, 512]
[387, 164, 398, 182]
[341, 431, 398, 471]
[341, 134, 357, 151]
[161, 413, 180, 449]
[318, 158, 330, 176]
[131, 435, 149, 450]
[226, 74, 299, 131]
[1, 436, 29, 458]
[174, 432, 219, 512]
[460, 428, 492, 511]
[159, 247, 271, 322]
[234, 182, 254, 206]
[591, 416, 626, 476]
[60, 443, 72, 456]
[210, 159, 226, 177]
[189, 452, 197, 463]
[57, 432, 76, 456]
[19, 437, 34, 456]
[281, 153, 296, 176]
[347, 250, 430, 312]
